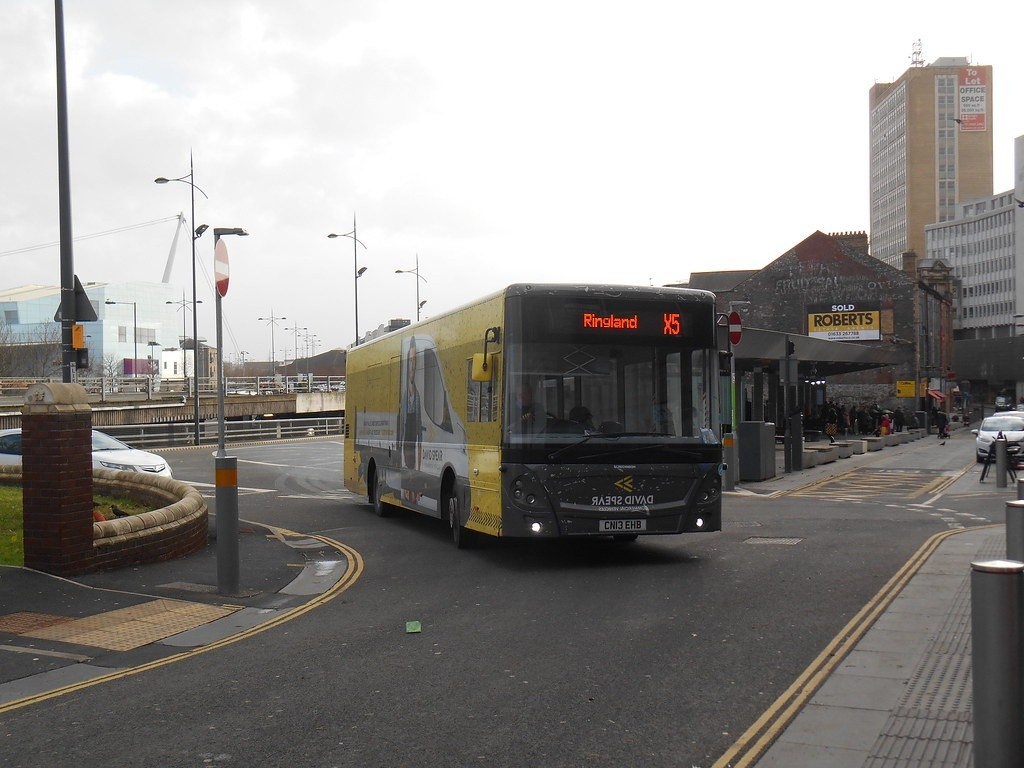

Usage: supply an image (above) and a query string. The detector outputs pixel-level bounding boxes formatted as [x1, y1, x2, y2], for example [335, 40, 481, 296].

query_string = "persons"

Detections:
[821, 400, 920, 443]
[507, 381, 557, 434]
[284, 384, 294, 392]
[399, 335, 422, 504]
[692, 407, 699, 436]
[961, 391, 970, 426]
[119, 388, 125, 393]
[931, 406, 947, 438]
[331, 385, 334, 390]
[94, 389, 98, 393]
[1016, 397, 1024, 412]
[259, 386, 263, 395]
[145, 383, 149, 393]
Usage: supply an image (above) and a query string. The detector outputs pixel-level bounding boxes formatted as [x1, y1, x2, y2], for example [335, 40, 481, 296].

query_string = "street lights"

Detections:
[327, 209, 368, 346]
[258, 307, 286, 386]
[155, 146, 209, 447]
[284, 321, 322, 376]
[105, 298, 138, 392]
[395, 252, 427, 321]
[147, 340, 160, 383]
[166, 288, 204, 392]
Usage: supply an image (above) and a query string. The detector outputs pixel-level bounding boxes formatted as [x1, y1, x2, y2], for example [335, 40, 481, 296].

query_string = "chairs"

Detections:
[600, 422, 623, 434]
[570, 406, 593, 429]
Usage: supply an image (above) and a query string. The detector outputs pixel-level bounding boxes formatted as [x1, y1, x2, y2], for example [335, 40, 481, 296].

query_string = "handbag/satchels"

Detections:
[825, 422, 837, 434]
[945, 424, 951, 432]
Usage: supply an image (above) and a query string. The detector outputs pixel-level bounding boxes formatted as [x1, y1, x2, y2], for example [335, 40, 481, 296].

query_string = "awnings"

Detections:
[929, 390, 948, 399]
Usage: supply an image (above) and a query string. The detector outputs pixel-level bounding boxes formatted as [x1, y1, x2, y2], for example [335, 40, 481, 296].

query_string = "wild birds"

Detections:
[1014, 197, 1024, 207]
[952, 118, 961, 123]
[940, 441, 945, 446]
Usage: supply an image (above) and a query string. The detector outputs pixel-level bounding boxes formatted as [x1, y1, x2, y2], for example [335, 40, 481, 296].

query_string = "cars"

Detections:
[971, 395, 1024, 462]
[0, 428, 175, 480]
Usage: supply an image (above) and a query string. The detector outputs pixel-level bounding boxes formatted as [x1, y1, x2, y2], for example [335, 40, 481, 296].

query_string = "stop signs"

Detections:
[214, 238, 230, 298]
[728, 311, 742, 346]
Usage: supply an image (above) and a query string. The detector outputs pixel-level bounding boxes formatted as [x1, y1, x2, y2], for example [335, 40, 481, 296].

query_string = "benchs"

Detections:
[802, 430, 821, 442]
[775, 436, 784, 444]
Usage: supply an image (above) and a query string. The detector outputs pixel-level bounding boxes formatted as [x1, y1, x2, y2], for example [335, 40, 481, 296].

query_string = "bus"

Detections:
[344, 284, 733, 550]
